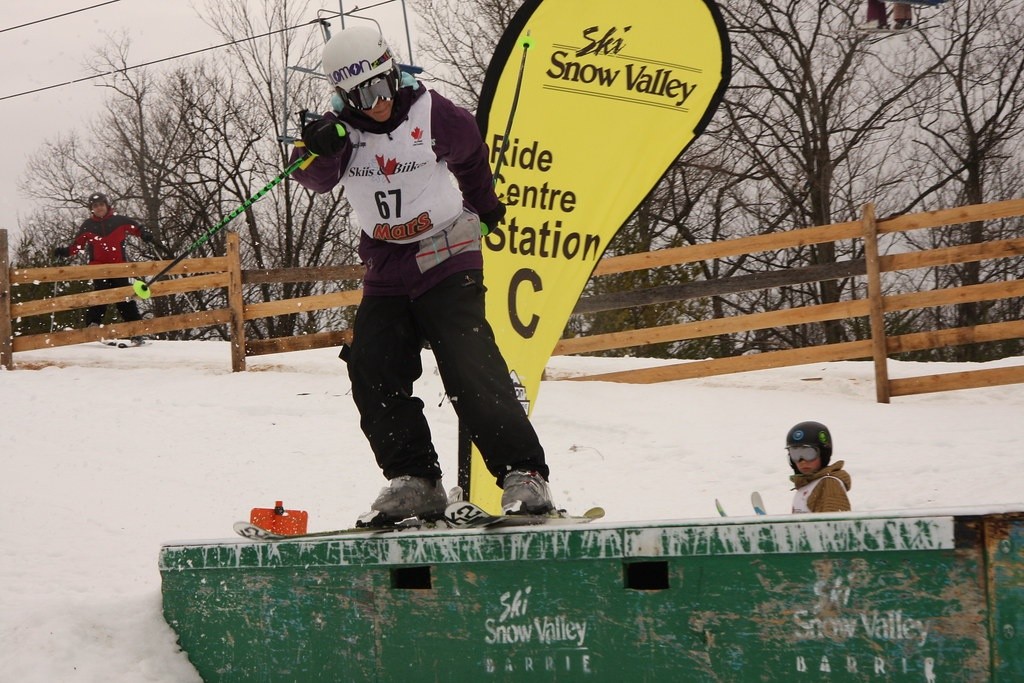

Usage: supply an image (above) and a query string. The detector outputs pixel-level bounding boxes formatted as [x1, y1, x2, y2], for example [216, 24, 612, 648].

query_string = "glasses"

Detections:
[338, 66, 398, 110]
[786, 447, 819, 462]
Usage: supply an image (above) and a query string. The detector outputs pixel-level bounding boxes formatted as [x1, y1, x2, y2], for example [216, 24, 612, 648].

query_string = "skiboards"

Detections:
[713, 492, 766, 516]
[64, 328, 152, 348]
[232, 505, 605, 541]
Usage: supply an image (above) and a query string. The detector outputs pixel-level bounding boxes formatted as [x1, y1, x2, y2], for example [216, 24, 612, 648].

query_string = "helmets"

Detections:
[324, 24, 395, 94]
[784, 421, 832, 457]
[89, 192, 106, 204]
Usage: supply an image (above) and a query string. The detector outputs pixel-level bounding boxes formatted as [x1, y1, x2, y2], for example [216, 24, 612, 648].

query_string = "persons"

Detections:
[785, 420, 852, 514]
[290, 26, 553, 516]
[55, 192, 154, 342]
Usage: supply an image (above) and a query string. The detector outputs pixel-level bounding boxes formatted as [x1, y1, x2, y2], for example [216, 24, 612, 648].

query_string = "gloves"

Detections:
[479, 202, 507, 237]
[142, 233, 153, 243]
[53, 247, 68, 257]
[301, 120, 348, 155]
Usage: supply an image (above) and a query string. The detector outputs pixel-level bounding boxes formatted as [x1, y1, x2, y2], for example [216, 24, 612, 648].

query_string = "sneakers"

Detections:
[373, 475, 448, 519]
[131, 336, 142, 343]
[88, 323, 100, 341]
[501, 469, 554, 516]
